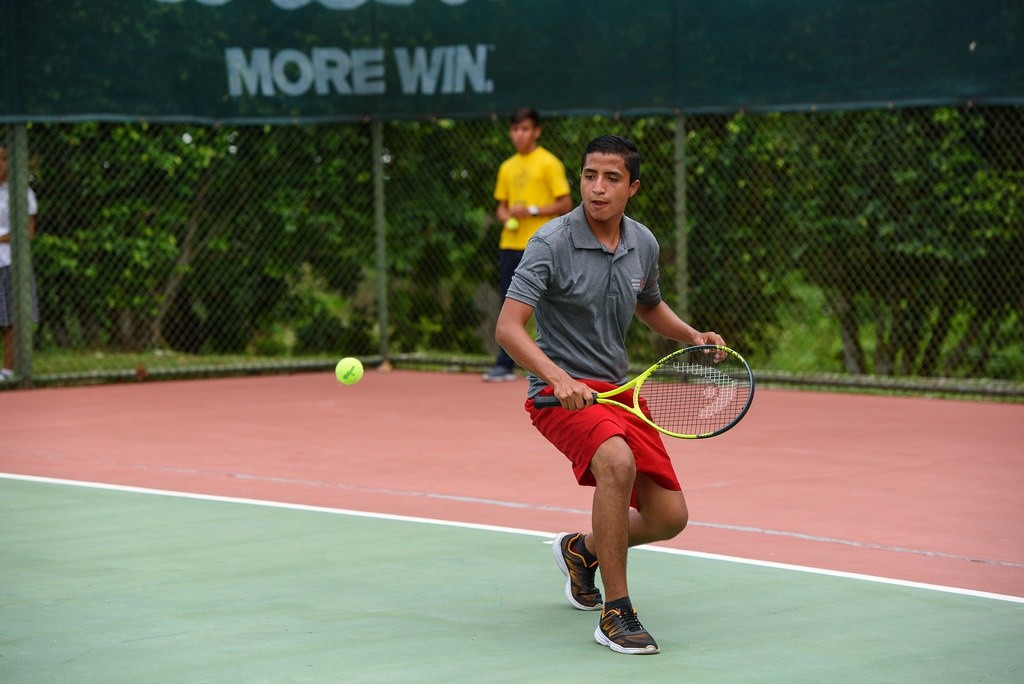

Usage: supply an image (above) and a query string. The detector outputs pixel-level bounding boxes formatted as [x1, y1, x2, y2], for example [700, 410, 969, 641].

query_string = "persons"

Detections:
[480, 107, 574, 382]
[0, 147, 39, 380]
[495, 133, 729, 654]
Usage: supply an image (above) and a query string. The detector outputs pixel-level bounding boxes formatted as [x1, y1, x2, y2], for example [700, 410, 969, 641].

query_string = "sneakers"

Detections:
[594, 608, 661, 655]
[553, 531, 604, 611]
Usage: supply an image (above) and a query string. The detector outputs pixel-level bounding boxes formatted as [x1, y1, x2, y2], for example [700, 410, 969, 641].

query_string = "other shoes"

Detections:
[482, 366, 516, 382]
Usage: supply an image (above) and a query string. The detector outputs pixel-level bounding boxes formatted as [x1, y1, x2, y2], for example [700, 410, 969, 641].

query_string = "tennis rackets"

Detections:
[534, 345, 755, 439]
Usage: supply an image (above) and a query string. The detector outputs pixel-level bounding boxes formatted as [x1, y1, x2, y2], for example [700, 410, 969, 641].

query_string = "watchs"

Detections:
[526, 205, 539, 215]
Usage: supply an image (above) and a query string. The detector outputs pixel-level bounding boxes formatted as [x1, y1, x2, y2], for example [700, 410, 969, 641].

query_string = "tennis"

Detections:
[505, 218, 520, 232]
[335, 357, 364, 385]
